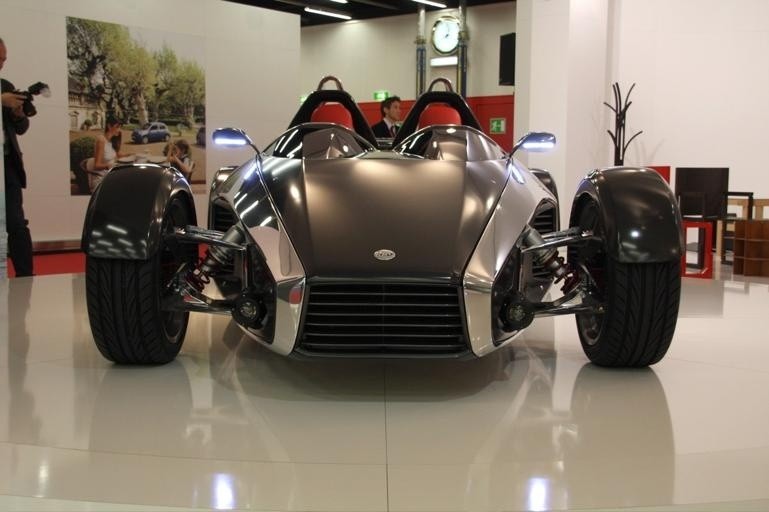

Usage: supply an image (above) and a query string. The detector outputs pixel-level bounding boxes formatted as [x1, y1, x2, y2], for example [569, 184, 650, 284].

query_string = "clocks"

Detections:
[430, 16, 460, 56]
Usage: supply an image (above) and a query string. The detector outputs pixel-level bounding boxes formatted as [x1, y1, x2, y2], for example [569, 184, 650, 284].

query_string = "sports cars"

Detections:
[82, 73, 682, 368]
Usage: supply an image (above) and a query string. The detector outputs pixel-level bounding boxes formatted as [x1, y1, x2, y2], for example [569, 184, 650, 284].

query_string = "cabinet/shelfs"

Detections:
[733, 220, 769, 276]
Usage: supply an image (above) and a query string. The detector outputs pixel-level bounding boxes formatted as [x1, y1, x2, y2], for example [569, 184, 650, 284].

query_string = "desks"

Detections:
[108, 154, 170, 169]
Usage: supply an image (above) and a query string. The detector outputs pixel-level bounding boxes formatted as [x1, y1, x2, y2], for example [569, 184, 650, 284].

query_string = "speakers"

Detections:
[499, 33, 515, 85]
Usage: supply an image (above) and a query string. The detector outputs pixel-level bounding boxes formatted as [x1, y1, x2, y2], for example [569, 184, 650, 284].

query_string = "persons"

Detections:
[371, 97, 401, 137]
[0, 39, 37, 276]
[167, 136, 192, 181]
[88, 115, 122, 190]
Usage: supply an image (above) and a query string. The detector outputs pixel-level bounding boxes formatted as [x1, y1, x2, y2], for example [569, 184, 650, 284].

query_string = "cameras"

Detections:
[13, 81, 50, 117]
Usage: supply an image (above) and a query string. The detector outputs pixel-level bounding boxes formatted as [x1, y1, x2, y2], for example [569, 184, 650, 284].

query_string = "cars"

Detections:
[131, 120, 172, 144]
[195, 126, 205, 147]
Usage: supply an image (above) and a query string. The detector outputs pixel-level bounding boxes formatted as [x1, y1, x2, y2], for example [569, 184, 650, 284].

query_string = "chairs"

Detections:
[80, 157, 105, 195]
[185, 161, 195, 184]
